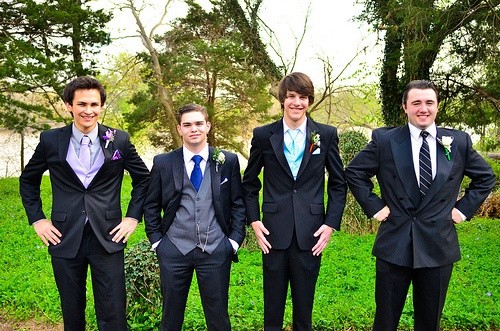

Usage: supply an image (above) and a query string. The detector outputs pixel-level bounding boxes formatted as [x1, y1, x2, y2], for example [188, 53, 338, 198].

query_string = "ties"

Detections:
[285, 129, 301, 158]
[418, 131, 433, 196]
[79, 137, 91, 172]
[190, 155, 203, 192]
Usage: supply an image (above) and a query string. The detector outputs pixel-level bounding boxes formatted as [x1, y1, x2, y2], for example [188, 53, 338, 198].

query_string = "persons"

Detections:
[19, 76, 151, 331]
[239, 72, 346, 331]
[141, 104, 247, 331]
[342, 79, 497, 331]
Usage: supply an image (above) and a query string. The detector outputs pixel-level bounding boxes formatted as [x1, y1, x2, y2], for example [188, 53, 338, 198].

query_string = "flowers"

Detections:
[212, 148, 225, 172]
[103, 129, 114, 148]
[441, 135, 453, 160]
[310, 131, 321, 152]
